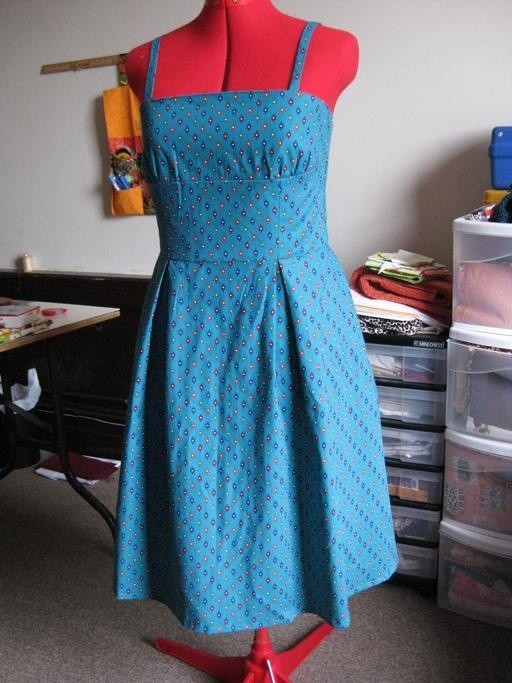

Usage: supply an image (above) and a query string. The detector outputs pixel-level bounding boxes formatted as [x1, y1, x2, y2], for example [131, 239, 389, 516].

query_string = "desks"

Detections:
[0, 299, 122, 558]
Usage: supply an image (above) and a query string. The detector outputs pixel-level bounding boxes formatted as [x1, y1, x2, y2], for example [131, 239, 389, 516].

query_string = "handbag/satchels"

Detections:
[102, 85, 156, 217]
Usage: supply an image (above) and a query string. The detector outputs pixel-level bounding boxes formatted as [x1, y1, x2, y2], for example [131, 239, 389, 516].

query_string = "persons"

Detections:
[113, 0, 400, 635]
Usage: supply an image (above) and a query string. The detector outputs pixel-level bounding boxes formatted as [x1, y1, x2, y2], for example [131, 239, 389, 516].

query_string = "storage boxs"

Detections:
[438, 521, 511, 632]
[451, 203, 511, 339]
[390, 493, 440, 545]
[367, 330, 450, 381]
[436, 428, 511, 543]
[377, 380, 447, 425]
[383, 454, 443, 507]
[382, 417, 445, 462]
[390, 535, 439, 596]
[445, 328, 511, 442]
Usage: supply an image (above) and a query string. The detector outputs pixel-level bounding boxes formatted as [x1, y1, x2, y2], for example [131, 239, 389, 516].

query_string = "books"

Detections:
[0, 305, 40, 329]
